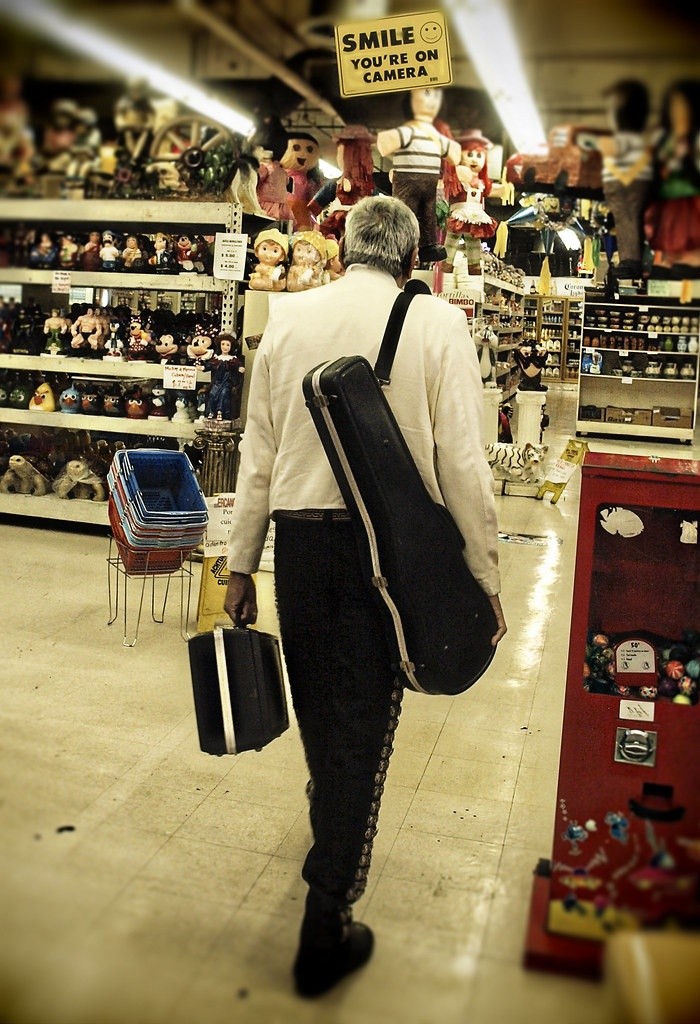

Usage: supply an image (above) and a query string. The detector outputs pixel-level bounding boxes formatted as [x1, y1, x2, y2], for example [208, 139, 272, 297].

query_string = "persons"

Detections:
[31, 229, 179, 274]
[377, 85, 462, 261]
[249, 121, 376, 238]
[437, 128, 497, 275]
[0, 297, 131, 350]
[576, 75, 700, 281]
[225, 193, 506, 997]
[250, 227, 344, 292]
[197, 332, 246, 420]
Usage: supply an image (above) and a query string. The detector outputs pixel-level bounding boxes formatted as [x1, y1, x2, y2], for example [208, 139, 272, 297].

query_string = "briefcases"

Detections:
[188, 603, 289, 756]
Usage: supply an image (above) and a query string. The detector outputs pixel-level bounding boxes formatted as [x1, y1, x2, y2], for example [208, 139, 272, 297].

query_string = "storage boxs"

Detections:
[579, 404, 693, 429]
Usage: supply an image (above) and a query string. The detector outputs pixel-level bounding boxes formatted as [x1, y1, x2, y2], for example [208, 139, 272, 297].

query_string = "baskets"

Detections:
[106, 448, 210, 575]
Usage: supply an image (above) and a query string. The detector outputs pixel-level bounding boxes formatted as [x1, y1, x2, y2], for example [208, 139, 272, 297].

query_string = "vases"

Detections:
[665, 337, 673, 352]
[677, 336, 687, 352]
[688, 337, 698, 353]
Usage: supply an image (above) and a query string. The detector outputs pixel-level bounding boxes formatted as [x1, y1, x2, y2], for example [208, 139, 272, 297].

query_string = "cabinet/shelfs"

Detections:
[456, 269, 584, 403]
[0, 200, 295, 562]
[575, 292, 700, 448]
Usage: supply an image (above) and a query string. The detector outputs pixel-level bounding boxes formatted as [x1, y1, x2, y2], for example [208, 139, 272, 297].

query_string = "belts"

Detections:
[278, 509, 354, 520]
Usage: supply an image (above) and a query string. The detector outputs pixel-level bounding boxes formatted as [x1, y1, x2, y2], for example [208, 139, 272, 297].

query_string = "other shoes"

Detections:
[292, 921, 374, 1000]
